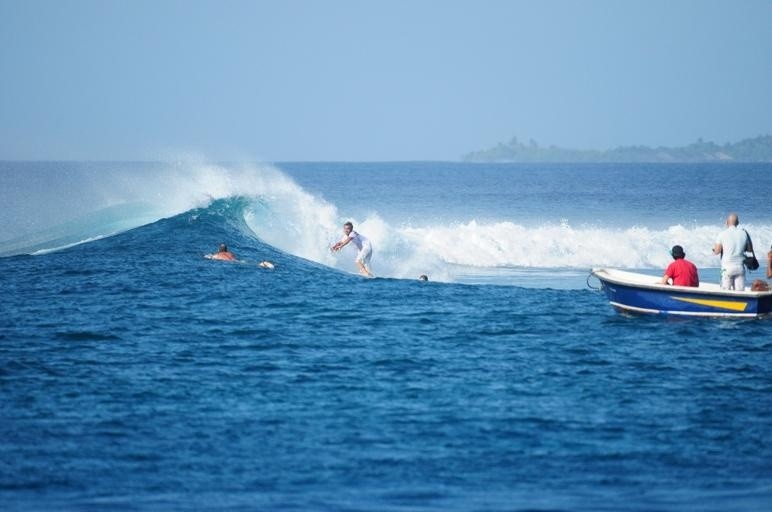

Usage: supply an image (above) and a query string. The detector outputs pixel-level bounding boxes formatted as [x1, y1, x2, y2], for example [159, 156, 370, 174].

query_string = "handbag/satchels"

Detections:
[743, 257, 759, 270]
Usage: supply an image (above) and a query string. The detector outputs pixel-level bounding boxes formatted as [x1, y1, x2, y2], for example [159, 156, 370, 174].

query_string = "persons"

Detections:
[330, 221, 374, 278]
[655, 245, 699, 288]
[209, 244, 275, 269]
[713, 213, 752, 291]
[419, 276, 428, 281]
[767, 245, 772, 279]
[750, 279, 768, 292]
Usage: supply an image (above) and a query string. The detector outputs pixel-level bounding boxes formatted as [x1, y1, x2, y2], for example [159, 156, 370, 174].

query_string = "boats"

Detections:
[587, 267, 772, 323]
